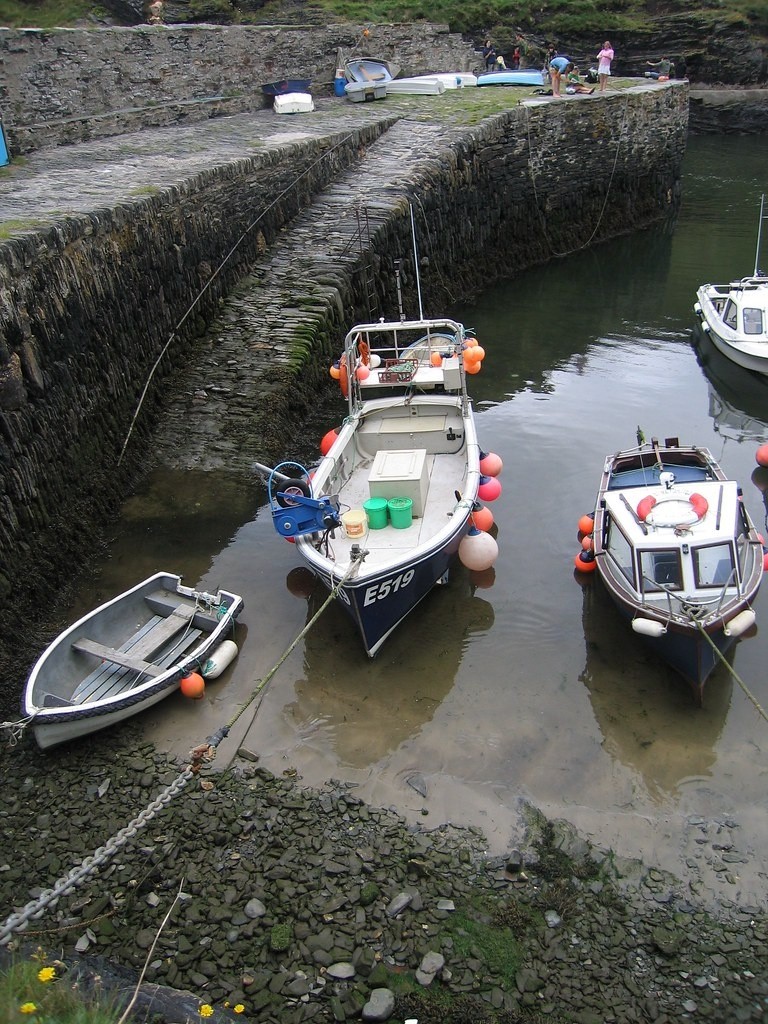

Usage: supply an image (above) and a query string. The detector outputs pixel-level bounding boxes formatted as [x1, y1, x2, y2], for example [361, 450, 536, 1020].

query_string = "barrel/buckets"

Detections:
[363, 496, 387, 529]
[388, 496, 413, 528]
[336, 69, 345, 77]
[334, 78, 348, 96]
[343, 510, 366, 539]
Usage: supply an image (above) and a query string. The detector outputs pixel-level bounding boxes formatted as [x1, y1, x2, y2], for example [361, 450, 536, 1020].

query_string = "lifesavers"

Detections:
[636, 490, 708, 529]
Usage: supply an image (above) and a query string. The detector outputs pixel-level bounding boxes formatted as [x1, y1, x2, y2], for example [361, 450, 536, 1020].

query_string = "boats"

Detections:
[23, 570, 248, 749]
[267, 205, 502, 657]
[574, 436, 765, 707]
[692, 194, 767, 378]
[260, 76, 316, 116]
[338, 58, 546, 103]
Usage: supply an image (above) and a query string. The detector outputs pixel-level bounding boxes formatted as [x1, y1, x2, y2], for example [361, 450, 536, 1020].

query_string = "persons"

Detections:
[510, 34, 528, 72]
[645, 54, 688, 80]
[596, 41, 614, 91]
[549, 57, 574, 99]
[566, 66, 597, 95]
[483, 40, 497, 72]
